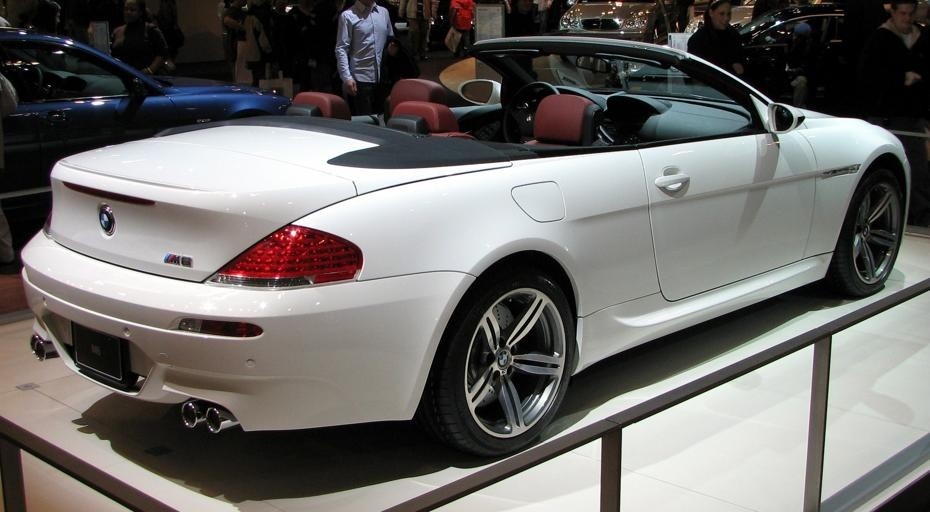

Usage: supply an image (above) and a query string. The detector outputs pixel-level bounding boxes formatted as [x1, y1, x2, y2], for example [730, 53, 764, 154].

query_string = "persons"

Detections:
[26, 0, 62, 71]
[504, 2, 569, 37]
[783, 21, 822, 110]
[221, 2, 397, 116]
[0, 74, 21, 265]
[113, 2, 178, 76]
[687, 0, 746, 81]
[869, 0, 930, 229]
[397, 1, 477, 63]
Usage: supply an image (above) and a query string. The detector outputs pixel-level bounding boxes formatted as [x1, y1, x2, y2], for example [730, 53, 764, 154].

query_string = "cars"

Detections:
[559, 0, 681, 44]
[684, 0, 930, 112]
[20, 37, 911, 459]
[0, 27, 293, 209]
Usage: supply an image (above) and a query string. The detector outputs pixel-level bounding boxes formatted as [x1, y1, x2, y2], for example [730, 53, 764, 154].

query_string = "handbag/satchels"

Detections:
[258, 79, 298, 101]
[445, 26, 462, 53]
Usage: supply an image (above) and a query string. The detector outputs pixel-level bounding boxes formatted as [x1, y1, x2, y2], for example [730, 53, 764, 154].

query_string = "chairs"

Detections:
[531, 92, 599, 144]
[292, 91, 356, 124]
[384, 75, 451, 125]
[391, 97, 480, 141]
